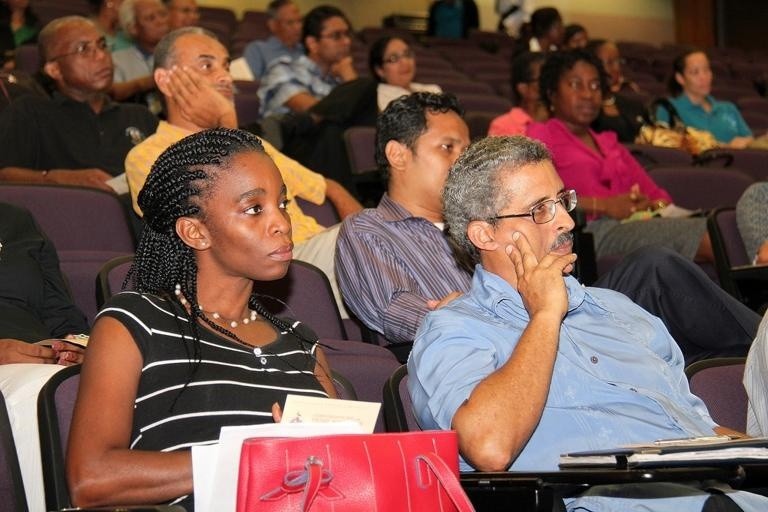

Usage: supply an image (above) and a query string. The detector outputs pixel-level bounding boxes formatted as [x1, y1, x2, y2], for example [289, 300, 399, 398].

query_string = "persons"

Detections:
[0, 0, 768, 367]
[65, 129, 336, 510]
[405, 136, 768, 511]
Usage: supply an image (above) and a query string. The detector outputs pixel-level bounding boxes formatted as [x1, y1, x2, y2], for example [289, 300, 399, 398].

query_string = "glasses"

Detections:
[384, 49, 415, 65]
[494, 187, 579, 225]
[48, 41, 115, 62]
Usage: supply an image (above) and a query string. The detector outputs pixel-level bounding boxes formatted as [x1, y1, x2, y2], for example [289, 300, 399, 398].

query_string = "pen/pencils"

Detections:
[653, 434, 732, 445]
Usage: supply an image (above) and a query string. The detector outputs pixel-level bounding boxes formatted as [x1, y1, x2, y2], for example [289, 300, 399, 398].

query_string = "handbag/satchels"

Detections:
[236, 428, 476, 512]
